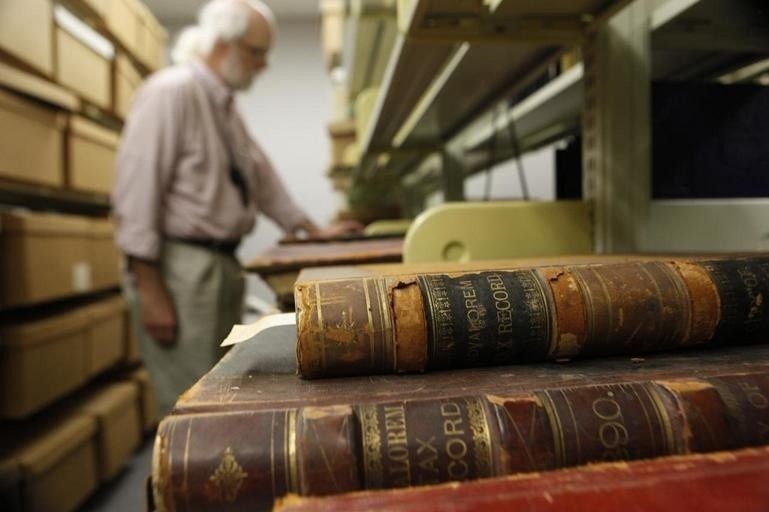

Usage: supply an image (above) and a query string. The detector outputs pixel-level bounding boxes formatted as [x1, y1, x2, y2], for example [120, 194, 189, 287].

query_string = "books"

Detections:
[152, 248, 769, 512]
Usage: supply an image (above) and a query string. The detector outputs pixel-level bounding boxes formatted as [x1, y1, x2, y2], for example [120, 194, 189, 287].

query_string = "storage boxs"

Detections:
[315, 0, 419, 186]
[0, 0, 179, 512]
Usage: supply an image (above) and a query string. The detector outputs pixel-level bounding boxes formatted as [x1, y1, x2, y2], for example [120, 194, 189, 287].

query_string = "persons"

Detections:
[108, 1, 329, 422]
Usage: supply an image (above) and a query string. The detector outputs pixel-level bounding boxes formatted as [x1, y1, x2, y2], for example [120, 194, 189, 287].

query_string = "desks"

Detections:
[246, 211, 404, 316]
